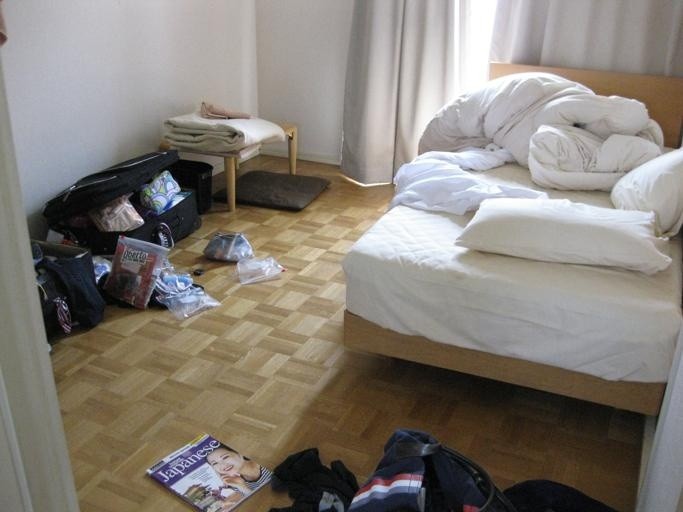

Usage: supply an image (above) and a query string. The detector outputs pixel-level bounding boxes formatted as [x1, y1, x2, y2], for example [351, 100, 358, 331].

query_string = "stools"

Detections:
[160, 121, 298, 210]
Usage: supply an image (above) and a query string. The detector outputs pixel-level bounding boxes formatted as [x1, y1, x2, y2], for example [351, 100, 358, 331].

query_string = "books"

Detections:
[145, 431, 274, 512]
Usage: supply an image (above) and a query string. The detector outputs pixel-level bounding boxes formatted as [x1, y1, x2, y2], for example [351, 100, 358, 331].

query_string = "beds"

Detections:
[342, 62, 680, 417]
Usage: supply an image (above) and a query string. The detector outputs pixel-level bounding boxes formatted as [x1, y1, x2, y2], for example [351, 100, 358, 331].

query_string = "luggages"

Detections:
[42, 149, 201, 257]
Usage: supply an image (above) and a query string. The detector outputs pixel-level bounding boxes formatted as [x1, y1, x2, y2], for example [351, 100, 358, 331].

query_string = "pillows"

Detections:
[452, 198, 672, 277]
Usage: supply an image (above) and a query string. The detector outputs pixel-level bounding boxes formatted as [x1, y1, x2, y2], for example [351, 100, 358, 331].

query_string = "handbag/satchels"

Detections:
[347, 429, 506, 511]
[203, 232, 253, 263]
